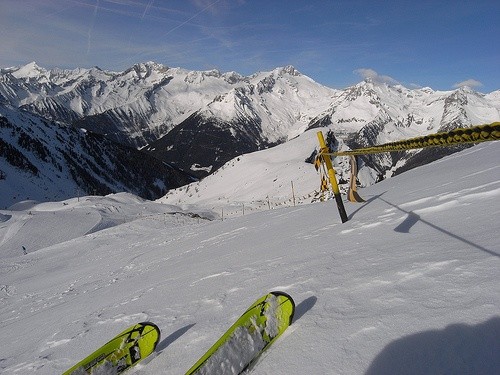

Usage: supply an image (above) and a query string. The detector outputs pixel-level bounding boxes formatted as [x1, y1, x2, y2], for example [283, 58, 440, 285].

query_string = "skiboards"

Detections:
[61, 288, 298, 375]
